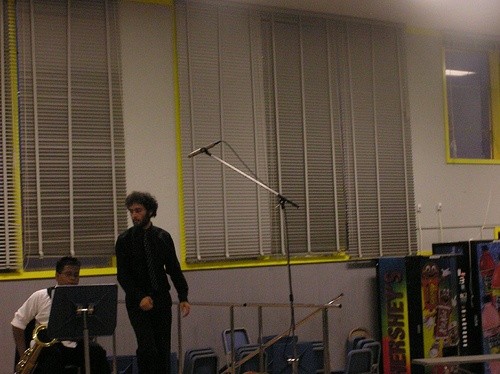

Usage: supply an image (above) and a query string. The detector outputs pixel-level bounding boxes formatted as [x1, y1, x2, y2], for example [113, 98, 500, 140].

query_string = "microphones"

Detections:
[188, 140, 221, 158]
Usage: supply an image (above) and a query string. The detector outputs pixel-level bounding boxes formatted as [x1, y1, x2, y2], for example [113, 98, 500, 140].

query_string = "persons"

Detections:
[11, 256, 108, 374]
[115, 192, 192, 374]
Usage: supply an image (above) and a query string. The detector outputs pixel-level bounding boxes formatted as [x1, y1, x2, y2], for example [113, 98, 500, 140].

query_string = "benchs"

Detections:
[412, 354, 500, 374]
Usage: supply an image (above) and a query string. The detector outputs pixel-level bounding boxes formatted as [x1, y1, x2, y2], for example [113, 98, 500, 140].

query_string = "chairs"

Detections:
[108, 327, 381, 374]
